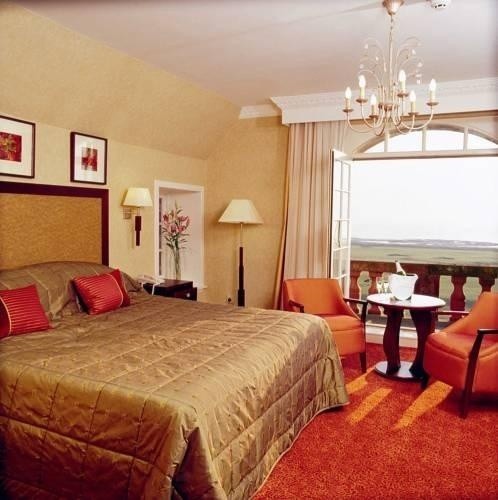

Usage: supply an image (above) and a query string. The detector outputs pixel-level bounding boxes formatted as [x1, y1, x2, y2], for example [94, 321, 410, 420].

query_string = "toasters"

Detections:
[375, 277, 383, 301]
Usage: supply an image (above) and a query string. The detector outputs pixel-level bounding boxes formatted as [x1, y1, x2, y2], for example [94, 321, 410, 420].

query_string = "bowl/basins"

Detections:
[388, 273, 419, 301]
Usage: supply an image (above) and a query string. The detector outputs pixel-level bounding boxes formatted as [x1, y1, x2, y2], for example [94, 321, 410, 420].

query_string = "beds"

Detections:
[1, 181, 350, 499]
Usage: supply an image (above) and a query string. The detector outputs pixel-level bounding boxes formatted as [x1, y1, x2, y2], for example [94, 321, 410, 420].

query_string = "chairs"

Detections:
[421, 290, 498, 419]
[283, 277, 368, 373]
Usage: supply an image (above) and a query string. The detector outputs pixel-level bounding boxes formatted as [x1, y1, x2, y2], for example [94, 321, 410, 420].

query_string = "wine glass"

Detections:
[381, 272, 389, 302]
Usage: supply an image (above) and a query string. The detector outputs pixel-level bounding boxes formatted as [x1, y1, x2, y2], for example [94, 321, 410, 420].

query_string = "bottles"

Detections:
[393, 260, 406, 276]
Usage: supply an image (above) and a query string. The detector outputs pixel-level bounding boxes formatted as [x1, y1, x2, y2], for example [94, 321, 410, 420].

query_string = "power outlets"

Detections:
[226, 295, 235, 304]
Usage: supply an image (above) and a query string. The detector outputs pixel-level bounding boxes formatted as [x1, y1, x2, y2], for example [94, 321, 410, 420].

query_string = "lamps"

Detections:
[121, 187, 154, 246]
[218, 198, 263, 306]
[343, 0, 439, 135]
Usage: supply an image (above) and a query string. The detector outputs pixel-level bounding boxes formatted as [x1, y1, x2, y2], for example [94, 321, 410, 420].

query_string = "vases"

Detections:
[174, 250, 182, 281]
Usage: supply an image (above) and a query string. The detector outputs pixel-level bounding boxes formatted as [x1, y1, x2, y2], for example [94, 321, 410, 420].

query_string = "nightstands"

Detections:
[137, 278, 198, 301]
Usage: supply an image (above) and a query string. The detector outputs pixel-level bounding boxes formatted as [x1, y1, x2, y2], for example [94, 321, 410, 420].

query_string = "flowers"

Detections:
[159, 201, 190, 273]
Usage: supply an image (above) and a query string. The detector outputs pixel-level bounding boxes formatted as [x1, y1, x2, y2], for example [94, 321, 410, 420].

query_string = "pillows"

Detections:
[72, 269, 137, 316]
[0, 284, 54, 339]
[314, 314, 363, 331]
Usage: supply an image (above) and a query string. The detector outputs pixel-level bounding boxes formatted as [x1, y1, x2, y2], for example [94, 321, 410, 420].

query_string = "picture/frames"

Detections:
[70, 131, 107, 184]
[0, 115, 36, 178]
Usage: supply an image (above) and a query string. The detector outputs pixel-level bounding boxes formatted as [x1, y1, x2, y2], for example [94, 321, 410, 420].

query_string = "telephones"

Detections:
[138, 274, 164, 284]
